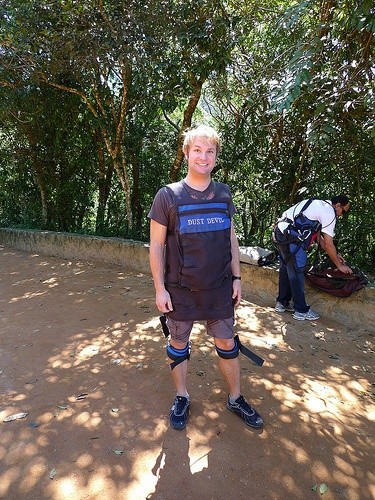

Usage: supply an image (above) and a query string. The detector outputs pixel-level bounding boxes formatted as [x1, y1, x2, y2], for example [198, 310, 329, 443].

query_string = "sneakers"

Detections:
[169, 391, 190, 430]
[275, 301, 295, 312]
[292, 308, 321, 321]
[226, 393, 264, 428]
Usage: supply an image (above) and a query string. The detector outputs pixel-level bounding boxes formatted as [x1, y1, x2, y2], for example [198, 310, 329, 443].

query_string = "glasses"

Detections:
[341, 205, 347, 214]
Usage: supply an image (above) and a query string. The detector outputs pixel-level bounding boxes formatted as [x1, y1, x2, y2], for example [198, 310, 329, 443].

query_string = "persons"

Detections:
[146, 123, 265, 430]
[271, 194, 353, 321]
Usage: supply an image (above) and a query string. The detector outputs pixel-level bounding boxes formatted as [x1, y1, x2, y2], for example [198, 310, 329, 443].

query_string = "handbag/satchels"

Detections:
[307, 265, 368, 298]
[283, 214, 322, 250]
